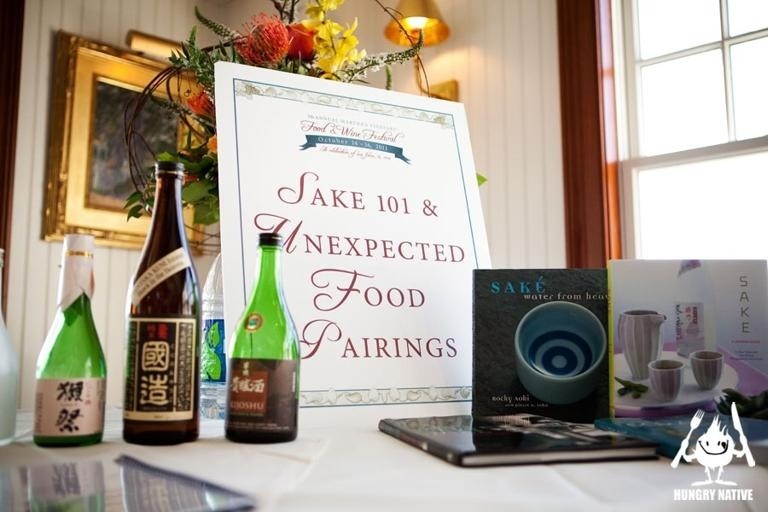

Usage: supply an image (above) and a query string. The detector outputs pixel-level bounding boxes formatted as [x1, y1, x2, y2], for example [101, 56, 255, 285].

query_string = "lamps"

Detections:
[383, 1, 460, 102]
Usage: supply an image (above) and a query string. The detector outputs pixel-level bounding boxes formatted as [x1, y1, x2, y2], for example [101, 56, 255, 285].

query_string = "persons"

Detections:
[91, 117, 123, 193]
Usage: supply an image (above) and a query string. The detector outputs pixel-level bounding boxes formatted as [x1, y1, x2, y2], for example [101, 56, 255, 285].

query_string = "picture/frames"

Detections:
[39, 28, 208, 252]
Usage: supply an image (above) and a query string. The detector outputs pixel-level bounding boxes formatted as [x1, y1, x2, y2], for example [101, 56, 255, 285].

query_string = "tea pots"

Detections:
[616, 308, 667, 381]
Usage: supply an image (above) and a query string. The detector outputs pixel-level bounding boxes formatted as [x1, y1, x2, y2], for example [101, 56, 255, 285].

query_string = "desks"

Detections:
[0, 403, 767, 512]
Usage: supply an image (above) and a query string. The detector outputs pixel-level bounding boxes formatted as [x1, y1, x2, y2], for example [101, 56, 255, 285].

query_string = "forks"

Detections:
[668, 407, 705, 469]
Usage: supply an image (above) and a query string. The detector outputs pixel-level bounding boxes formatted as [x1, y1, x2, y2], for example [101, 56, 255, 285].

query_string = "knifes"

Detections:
[729, 401, 756, 468]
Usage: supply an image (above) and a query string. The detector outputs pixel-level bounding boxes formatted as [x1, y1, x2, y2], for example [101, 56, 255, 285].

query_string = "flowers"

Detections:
[121, 1, 489, 228]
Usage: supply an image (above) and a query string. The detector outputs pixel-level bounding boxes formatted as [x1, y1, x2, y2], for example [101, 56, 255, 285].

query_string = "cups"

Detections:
[647, 358, 686, 403]
[689, 349, 724, 390]
[514, 300, 607, 406]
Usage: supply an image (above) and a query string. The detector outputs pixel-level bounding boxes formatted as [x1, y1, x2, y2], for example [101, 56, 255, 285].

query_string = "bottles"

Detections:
[121, 161, 201, 446]
[225, 232, 302, 446]
[32, 233, 103, 449]
[201, 250, 223, 420]
